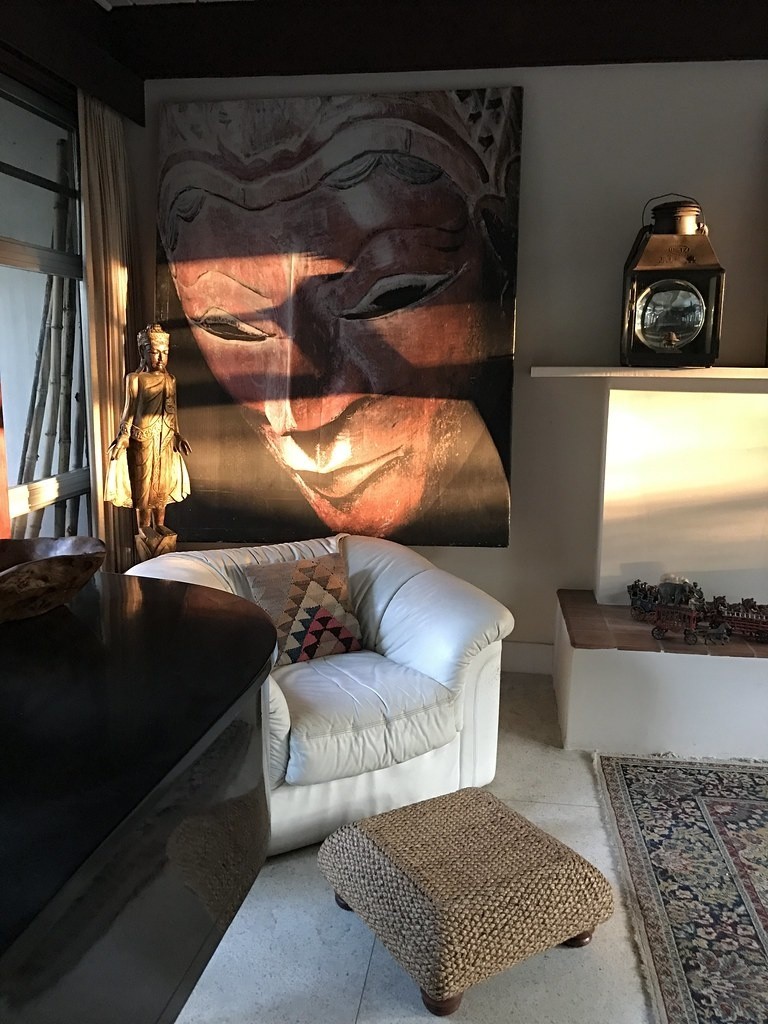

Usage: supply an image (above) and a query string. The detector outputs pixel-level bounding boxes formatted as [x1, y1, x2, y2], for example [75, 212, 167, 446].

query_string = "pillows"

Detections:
[244, 554, 363, 669]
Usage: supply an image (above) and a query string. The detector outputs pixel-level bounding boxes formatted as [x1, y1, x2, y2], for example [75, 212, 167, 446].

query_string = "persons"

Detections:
[675, 578, 703, 604]
[632, 579, 660, 604]
[688, 593, 700, 631]
[105, 326, 191, 550]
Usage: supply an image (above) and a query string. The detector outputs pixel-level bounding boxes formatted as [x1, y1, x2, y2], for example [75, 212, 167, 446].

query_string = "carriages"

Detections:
[624, 575, 768, 646]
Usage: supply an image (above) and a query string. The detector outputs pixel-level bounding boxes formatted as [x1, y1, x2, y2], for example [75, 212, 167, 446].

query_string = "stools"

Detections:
[316, 786, 614, 1017]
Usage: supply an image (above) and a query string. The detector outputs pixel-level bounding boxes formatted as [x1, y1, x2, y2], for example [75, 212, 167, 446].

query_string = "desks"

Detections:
[1, 574, 278, 1023]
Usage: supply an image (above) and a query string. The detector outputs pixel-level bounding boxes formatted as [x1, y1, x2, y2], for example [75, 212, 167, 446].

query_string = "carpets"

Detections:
[592, 751, 768, 1024]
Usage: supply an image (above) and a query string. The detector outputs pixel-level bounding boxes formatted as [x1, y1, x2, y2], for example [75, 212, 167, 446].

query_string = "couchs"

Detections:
[124, 532, 515, 856]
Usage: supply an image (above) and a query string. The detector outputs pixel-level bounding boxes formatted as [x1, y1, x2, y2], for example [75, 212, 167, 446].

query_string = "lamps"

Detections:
[619, 193, 726, 368]
